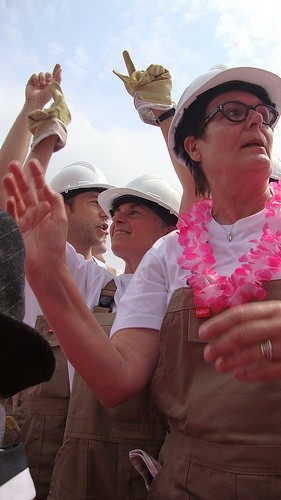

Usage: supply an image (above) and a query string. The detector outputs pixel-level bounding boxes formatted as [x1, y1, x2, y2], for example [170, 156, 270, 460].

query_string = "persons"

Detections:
[17, 50, 216, 499]
[3, 63, 281, 499]
[0, 63, 122, 500]
[88, 234, 108, 263]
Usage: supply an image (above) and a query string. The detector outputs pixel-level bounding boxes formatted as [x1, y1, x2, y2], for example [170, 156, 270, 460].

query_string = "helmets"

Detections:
[97, 174, 183, 220]
[168, 63, 281, 167]
[49, 162, 117, 194]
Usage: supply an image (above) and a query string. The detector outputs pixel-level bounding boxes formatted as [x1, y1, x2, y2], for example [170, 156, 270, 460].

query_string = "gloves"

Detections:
[27, 80, 71, 153]
[113, 50, 179, 126]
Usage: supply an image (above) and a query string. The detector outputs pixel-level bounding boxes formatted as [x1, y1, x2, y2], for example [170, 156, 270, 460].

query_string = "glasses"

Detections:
[200, 100, 279, 127]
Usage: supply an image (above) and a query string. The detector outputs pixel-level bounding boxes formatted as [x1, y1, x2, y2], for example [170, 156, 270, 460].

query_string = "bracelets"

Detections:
[154, 103, 177, 128]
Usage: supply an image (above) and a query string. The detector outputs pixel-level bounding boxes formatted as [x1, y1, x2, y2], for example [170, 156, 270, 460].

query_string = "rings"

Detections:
[258, 339, 272, 362]
